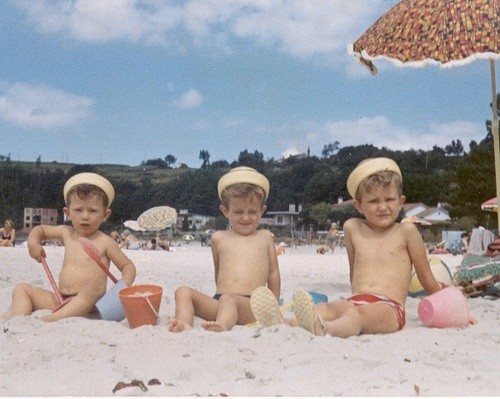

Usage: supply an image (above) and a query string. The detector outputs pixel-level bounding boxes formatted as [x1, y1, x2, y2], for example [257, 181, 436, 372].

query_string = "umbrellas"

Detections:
[182, 234, 194, 240]
[205, 229, 216, 235]
[348, 0, 500, 236]
[124, 206, 177, 231]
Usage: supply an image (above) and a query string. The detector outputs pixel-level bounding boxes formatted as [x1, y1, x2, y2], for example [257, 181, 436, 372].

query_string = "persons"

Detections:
[327, 222, 338, 253]
[111, 229, 173, 250]
[168, 166, 281, 333]
[250, 157, 478, 339]
[0, 219, 15, 247]
[0, 172, 137, 321]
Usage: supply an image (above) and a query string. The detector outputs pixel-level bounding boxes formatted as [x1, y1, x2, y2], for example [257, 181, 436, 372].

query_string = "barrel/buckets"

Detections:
[417, 286, 470, 328]
[118, 284, 163, 329]
[93, 279, 128, 323]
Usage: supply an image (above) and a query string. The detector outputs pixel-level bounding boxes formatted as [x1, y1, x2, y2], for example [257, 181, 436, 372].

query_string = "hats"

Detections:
[217, 166, 271, 203]
[63, 172, 116, 209]
[347, 156, 403, 200]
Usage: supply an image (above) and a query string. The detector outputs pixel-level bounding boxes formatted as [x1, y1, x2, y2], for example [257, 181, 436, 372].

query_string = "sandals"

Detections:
[292, 290, 327, 337]
[251, 286, 287, 326]
